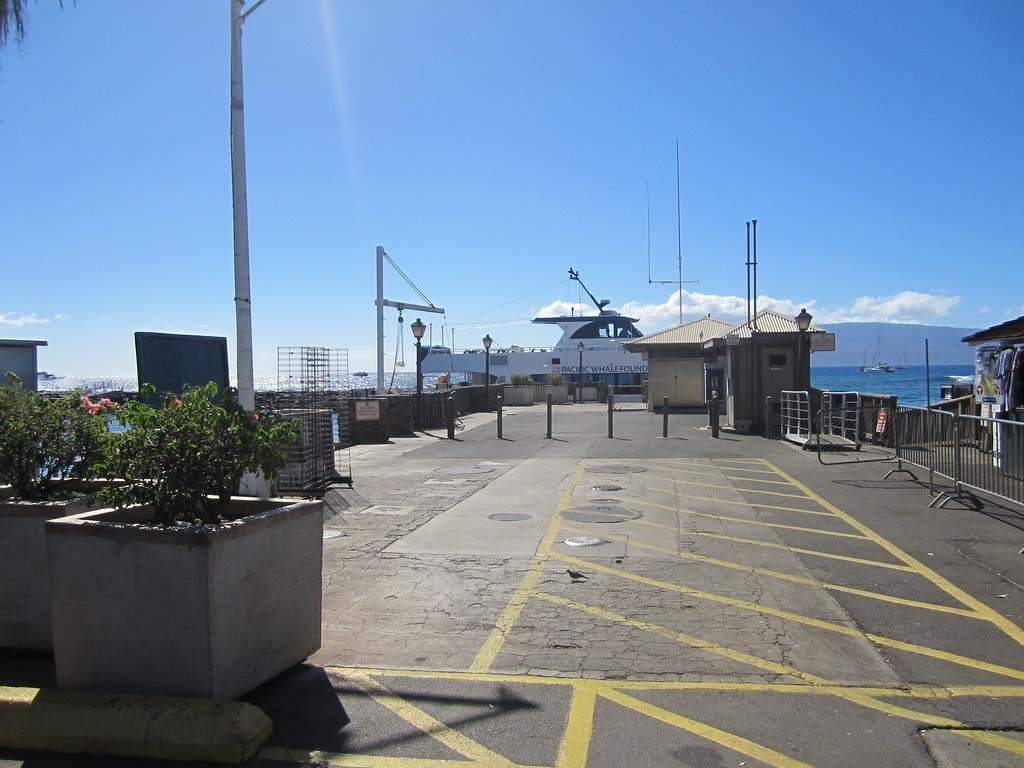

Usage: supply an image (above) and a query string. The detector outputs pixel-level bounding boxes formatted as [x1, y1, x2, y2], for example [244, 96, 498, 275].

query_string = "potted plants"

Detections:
[545, 373, 568, 403]
[0, 370, 323, 701]
[504, 374, 535, 405]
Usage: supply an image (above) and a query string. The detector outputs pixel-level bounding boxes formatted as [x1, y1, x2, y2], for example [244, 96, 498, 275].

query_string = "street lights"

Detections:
[482, 334, 493, 413]
[577, 341, 586, 403]
[410, 317, 428, 433]
[794, 307, 813, 433]
